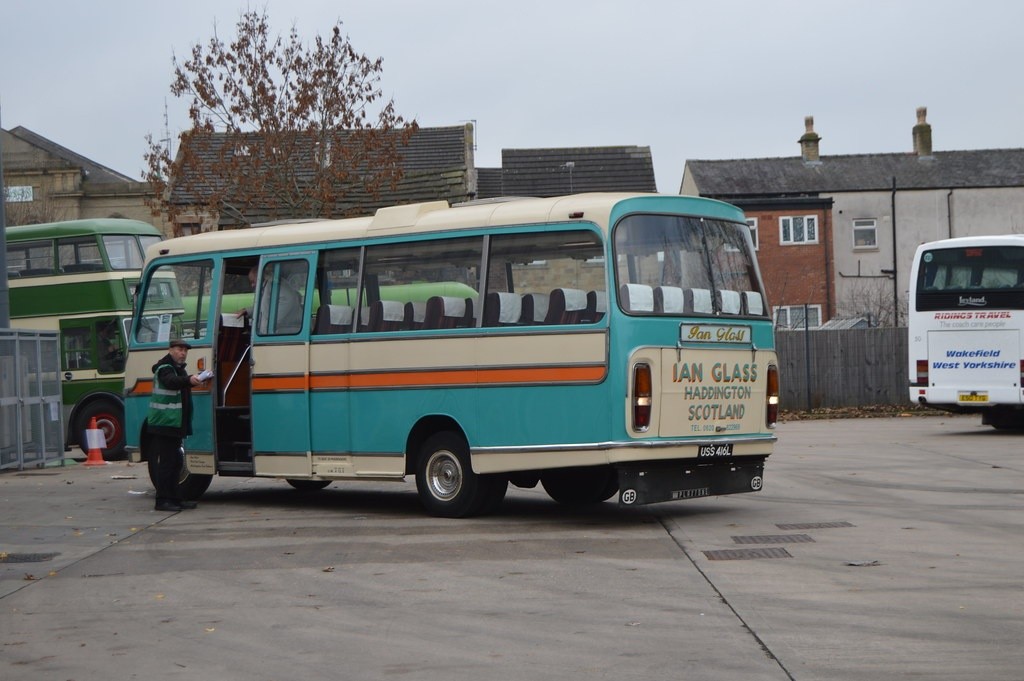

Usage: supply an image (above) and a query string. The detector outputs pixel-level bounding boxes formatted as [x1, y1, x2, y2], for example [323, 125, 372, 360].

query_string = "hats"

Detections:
[170, 340, 192, 349]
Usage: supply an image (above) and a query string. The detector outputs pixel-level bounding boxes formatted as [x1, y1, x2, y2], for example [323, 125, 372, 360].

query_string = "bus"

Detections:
[908, 233, 1024, 429]
[4, 218, 186, 463]
[124, 189, 782, 518]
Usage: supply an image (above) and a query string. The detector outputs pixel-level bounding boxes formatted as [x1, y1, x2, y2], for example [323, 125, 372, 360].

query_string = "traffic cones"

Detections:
[82, 416, 108, 465]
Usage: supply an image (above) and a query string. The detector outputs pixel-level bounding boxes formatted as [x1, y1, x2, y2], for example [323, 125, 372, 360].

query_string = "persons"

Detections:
[146, 339, 202, 512]
[235, 265, 304, 335]
[80, 328, 120, 369]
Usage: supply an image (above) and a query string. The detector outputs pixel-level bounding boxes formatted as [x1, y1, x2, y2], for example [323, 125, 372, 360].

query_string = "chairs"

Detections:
[212, 283, 765, 396]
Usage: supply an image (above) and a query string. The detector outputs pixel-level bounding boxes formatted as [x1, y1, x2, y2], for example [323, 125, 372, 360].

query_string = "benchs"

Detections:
[8, 263, 114, 280]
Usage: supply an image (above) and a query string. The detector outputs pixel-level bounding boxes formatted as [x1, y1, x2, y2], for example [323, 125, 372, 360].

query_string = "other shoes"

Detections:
[155, 503, 183, 511]
[179, 500, 197, 509]
